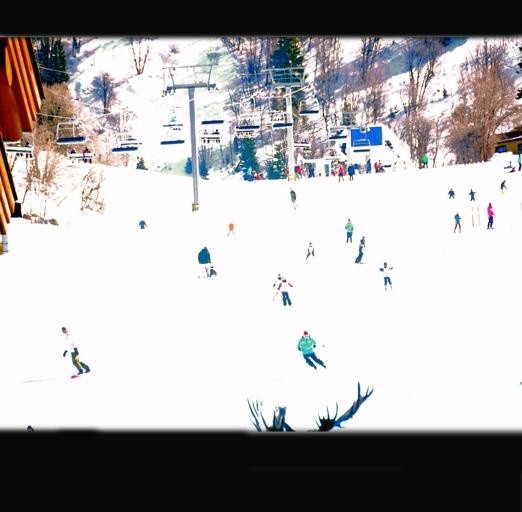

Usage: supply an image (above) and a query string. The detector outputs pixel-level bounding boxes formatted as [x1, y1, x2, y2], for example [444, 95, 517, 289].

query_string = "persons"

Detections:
[15, 140, 33, 158]
[274, 273, 295, 306]
[518, 152, 522, 171]
[355, 236, 366, 263]
[420, 153, 428, 168]
[298, 331, 326, 370]
[203, 128, 221, 144]
[306, 241, 314, 259]
[294, 158, 385, 182]
[448, 187, 456, 199]
[380, 262, 393, 288]
[504, 160, 517, 173]
[289, 186, 298, 210]
[197, 246, 216, 279]
[488, 202, 496, 229]
[345, 218, 354, 243]
[61, 326, 89, 375]
[70, 145, 91, 164]
[454, 213, 461, 232]
